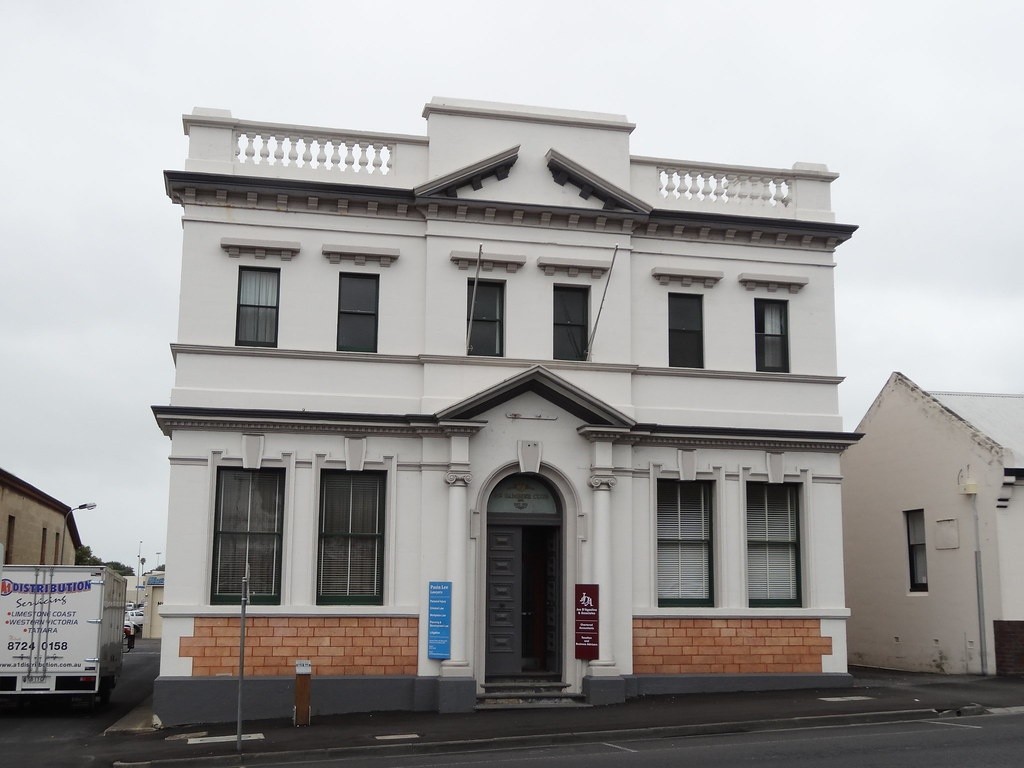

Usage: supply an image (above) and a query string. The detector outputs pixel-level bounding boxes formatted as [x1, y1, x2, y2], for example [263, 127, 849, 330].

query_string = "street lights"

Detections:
[60, 502, 97, 565]
[136, 540, 142, 610]
[156, 552, 162, 565]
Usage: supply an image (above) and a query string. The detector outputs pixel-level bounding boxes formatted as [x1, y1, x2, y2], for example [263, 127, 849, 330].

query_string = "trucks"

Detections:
[0, 565, 135, 711]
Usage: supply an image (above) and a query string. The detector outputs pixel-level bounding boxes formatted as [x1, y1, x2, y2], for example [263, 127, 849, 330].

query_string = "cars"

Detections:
[123, 620, 140, 639]
[125, 611, 144, 626]
[125, 603, 144, 612]
